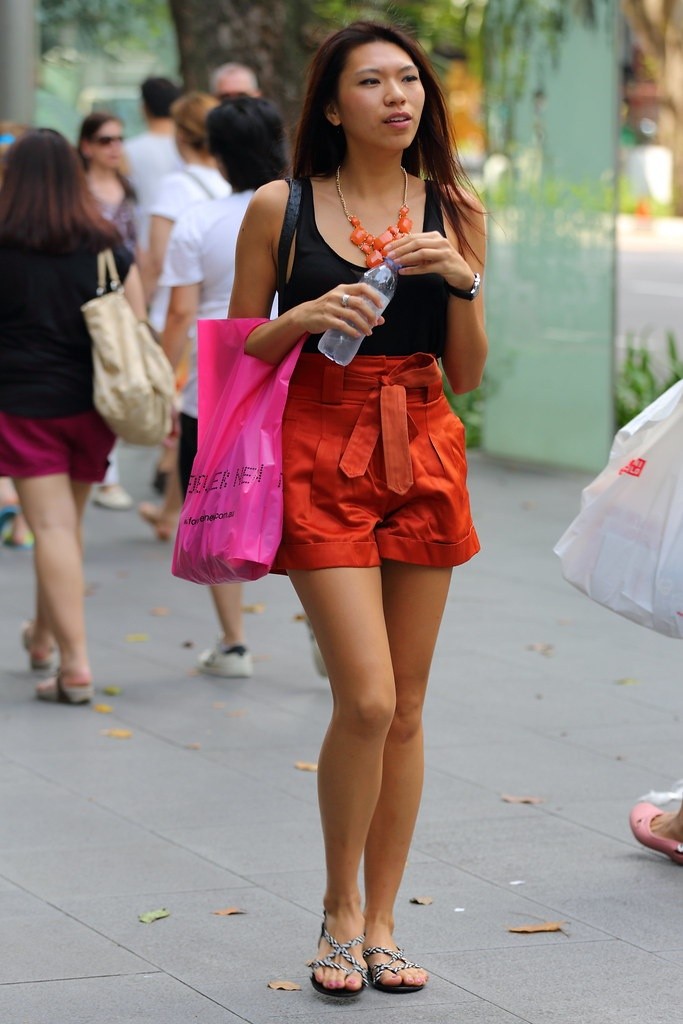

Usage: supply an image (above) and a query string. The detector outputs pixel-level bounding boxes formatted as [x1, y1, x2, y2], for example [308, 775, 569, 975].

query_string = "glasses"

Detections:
[87, 134, 124, 146]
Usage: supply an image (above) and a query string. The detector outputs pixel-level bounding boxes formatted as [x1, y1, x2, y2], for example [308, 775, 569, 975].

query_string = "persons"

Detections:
[0, 127, 146, 706]
[69, 112, 144, 511]
[630, 795, 683, 864]
[208, 60, 263, 103]
[119, 76, 183, 340]
[139, 93, 231, 541]
[214, 21, 489, 997]
[154, 94, 330, 681]
[1, 471, 36, 550]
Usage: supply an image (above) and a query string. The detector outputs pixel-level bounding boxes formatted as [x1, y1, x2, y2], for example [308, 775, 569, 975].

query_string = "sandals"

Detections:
[362, 946, 425, 994]
[307, 909, 369, 997]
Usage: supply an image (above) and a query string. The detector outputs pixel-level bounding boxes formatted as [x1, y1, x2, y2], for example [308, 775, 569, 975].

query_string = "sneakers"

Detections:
[313, 639, 329, 678]
[198, 643, 254, 678]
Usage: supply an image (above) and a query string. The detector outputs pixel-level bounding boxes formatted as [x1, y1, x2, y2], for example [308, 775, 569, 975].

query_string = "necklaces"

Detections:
[336, 163, 413, 269]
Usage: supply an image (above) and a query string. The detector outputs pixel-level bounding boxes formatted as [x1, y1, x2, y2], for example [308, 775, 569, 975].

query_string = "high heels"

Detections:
[22, 622, 53, 670]
[36, 666, 94, 705]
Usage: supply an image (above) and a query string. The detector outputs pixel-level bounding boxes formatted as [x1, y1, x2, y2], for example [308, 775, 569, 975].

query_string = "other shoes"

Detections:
[0, 505, 22, 533]
[151, 466, 173, 497]
[95, 487, 134, 511]
[628, 801, 683, 864]
[141, 500, 171, 540]
[1, 521, 36, 550]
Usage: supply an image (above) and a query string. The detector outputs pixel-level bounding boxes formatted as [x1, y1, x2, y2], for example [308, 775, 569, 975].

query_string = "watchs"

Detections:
[451, 272, 481, 301]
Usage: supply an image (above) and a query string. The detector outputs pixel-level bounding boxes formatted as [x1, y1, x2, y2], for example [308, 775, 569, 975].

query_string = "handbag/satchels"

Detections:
[79, 248, 175, 444]
[553, 378, 682, 641]
[170, 316, 309, 586]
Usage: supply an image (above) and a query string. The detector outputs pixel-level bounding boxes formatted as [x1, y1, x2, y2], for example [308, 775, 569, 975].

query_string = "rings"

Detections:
[341, 295, 350, 305]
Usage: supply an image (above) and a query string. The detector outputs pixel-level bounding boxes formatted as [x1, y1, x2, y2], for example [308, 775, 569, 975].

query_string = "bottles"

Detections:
[317, 255, 402, 366]
